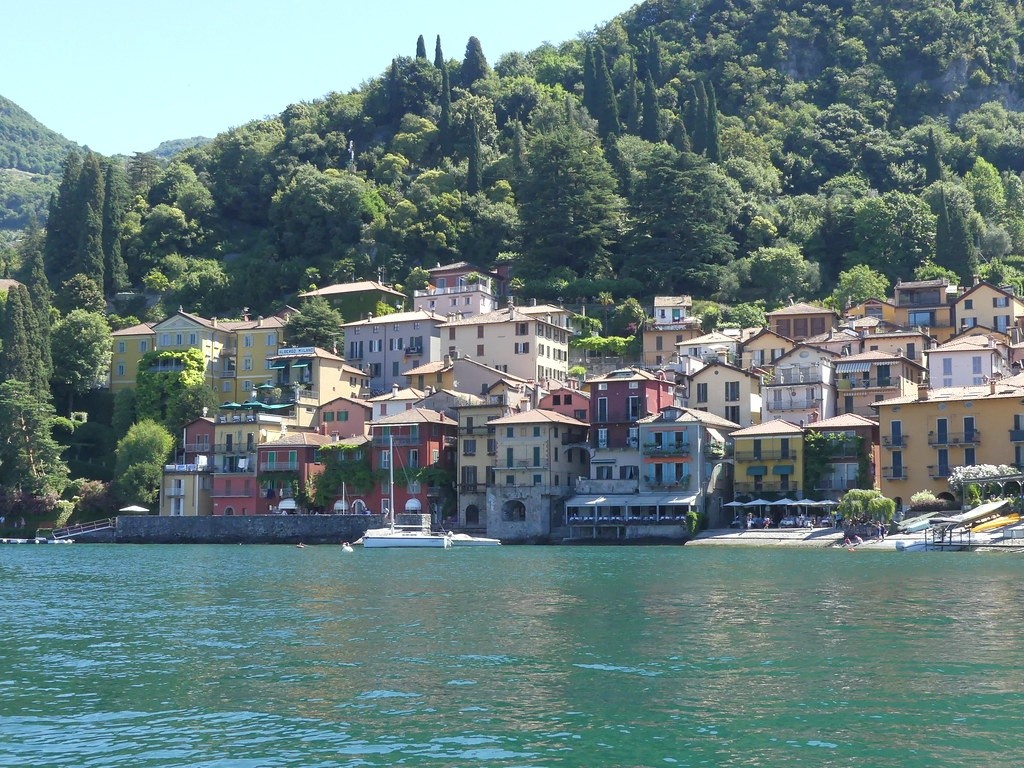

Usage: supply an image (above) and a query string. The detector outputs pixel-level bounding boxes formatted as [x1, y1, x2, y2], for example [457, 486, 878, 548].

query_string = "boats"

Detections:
[896, 538, 992, 552]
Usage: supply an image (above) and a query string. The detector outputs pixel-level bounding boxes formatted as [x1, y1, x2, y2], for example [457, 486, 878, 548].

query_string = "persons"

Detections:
[363, 507, 368, 514]
[747, 513, 752, 530]
[835, 512, 843, 530]
[764, 515, 770, 529]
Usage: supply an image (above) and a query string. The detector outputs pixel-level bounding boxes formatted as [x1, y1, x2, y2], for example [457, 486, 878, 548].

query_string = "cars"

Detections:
[730, 517, 744, 528]
[780, 517, 797, 527]
[821, 515, 831, 527]
[750, 515, 777, 528]
[803, 518, 814, 527]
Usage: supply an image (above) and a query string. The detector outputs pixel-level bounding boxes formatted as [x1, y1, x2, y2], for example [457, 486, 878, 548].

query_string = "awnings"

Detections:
[591, 459, 616, 463]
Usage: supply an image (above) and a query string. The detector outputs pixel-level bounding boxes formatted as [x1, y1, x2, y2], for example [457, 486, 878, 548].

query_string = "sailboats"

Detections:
[363, 434, 502, 547]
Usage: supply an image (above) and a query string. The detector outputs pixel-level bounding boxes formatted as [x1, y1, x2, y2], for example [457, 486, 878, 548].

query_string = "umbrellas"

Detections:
[720, 498, 841, 519]
[119, 505, 150, 515]
